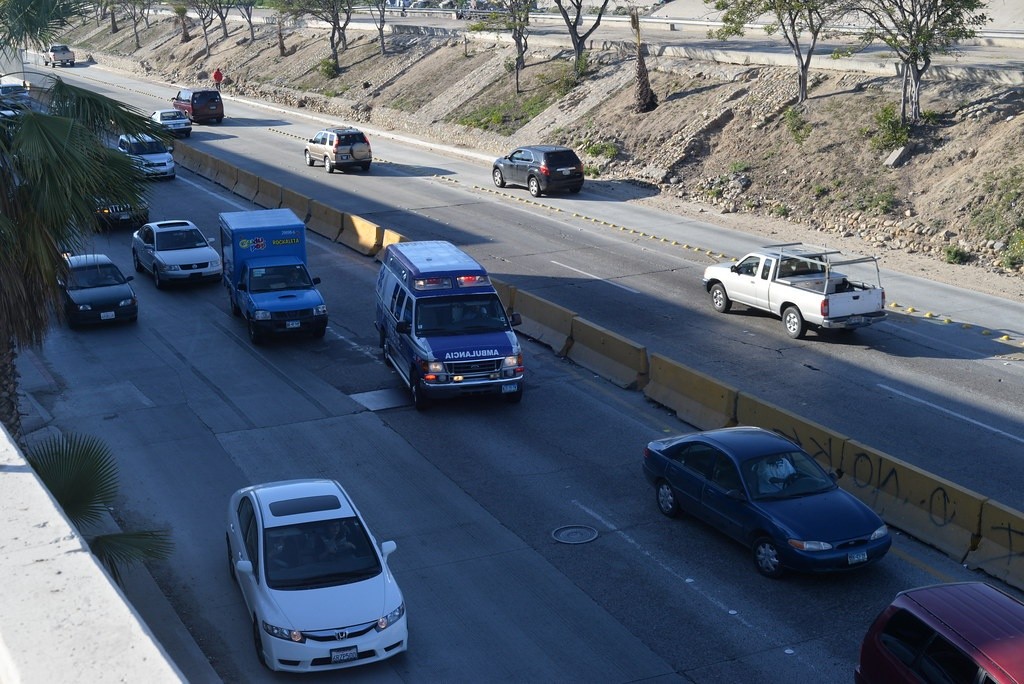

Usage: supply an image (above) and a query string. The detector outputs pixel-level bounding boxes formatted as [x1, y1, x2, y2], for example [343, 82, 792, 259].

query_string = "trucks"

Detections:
[218, 208, 330, 346]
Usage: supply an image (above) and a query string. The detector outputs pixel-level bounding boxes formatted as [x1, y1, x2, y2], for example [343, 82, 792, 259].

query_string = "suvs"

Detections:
[119, 134, 175, 182]
[492, 145, 585, 197]
[304, 126, 373, 174]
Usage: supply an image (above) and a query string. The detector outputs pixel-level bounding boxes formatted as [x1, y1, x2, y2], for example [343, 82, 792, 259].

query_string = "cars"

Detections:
[638, 424, 891, 580]
[149, 110, 193, 138]
[132, 221, 225, 290]
[89, 186, 150, 235]
[57, 254, 139, 332]
[0, 74, 74, 144]
[224, 477, 409, 676]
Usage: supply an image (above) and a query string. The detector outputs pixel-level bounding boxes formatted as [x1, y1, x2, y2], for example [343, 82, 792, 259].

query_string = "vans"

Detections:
[856, 579, 1024, 684]
[171, 87, 224, 125]
[373, 240, 524, 413]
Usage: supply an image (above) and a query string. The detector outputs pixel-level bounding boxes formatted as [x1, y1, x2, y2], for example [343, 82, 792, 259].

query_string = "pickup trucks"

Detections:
[43, 42, 76, 69]
[702, 243, 889, 340]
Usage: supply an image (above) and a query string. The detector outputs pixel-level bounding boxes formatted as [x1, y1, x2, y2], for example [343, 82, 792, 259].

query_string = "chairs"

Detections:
[795, 262, 810, 271]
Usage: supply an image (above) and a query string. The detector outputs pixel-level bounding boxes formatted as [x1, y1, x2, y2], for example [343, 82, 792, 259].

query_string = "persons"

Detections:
[270, 269, 287, 288]
[314, 520, 356, 562]
[756, 449, 798, 495]
[266, 532, 298, 572]
[95, 266, 113, 286]
[213, 68, 222, 93]
